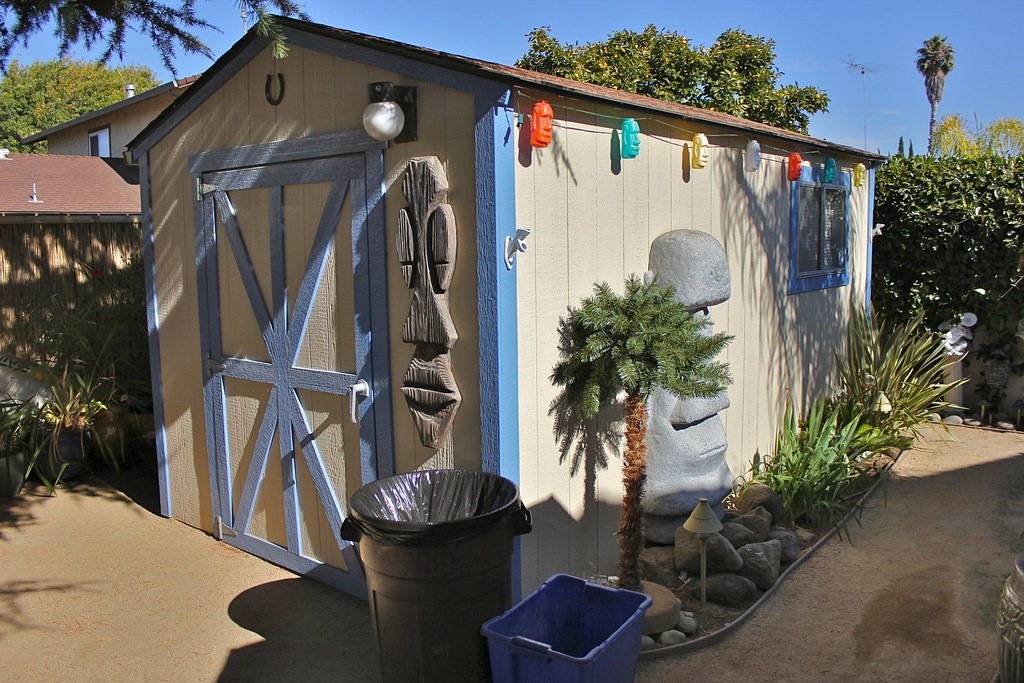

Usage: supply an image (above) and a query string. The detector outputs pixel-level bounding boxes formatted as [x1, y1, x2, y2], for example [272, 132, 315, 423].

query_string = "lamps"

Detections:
[361, 82, 418, 145]
[683, 498, 723, 541]
[870, 390, 892, 415]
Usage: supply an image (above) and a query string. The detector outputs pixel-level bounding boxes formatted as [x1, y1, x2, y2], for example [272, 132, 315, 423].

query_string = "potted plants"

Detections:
[21, 293, 136, 462]
[15, 357, 113, 495]
[0, 394, 37, 496]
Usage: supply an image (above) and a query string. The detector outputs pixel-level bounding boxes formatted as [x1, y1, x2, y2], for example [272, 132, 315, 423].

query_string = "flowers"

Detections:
[70, 251, 154, 394]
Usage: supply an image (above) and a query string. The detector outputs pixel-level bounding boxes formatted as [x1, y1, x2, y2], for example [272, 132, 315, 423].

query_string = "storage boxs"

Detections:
[480, 573, 653, 683]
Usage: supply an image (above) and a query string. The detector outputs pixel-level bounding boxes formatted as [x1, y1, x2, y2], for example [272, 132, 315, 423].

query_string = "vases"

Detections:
[109, 381, 157, 461]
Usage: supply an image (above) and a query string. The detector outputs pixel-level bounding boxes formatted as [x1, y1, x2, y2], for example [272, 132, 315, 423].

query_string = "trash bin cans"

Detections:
[343, 471, 522, 683]
